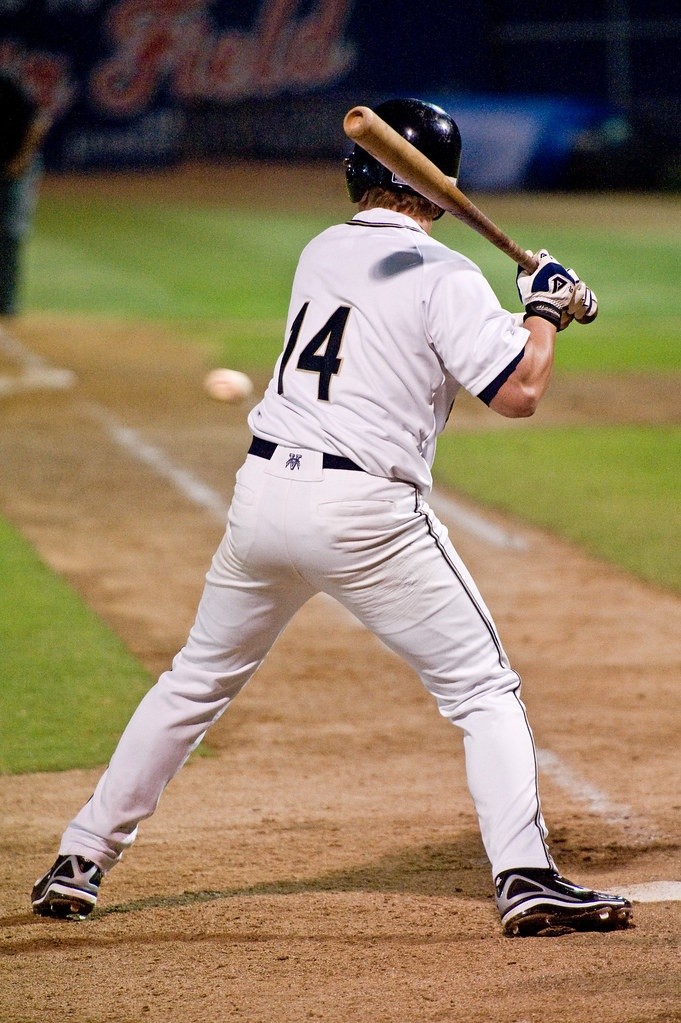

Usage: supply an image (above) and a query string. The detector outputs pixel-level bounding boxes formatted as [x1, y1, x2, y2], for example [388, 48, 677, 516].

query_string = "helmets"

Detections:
[344, 97, 462, 220]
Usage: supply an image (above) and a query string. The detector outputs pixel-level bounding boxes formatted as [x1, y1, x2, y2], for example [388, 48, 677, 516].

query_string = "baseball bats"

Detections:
[342, 105, 599, 325]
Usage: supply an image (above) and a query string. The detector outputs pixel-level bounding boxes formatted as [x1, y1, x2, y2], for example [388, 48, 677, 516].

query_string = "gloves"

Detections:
[516, 249, 600, 331]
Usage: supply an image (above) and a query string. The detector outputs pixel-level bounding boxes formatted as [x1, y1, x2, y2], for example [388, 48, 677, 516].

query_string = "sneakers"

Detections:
[493, 869, 633, 936]
[31, 854, 103, 917]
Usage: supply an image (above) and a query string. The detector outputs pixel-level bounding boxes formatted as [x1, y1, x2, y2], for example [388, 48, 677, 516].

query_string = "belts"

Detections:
[247, 435, 365, 472]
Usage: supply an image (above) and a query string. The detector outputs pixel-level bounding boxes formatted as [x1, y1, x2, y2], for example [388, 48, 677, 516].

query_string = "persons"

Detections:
[0, 38, 67, 316]
[30, 96, 638, 935]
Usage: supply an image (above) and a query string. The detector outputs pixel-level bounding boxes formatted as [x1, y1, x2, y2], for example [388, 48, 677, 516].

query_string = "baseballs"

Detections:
[205, 367, 254, 404]
